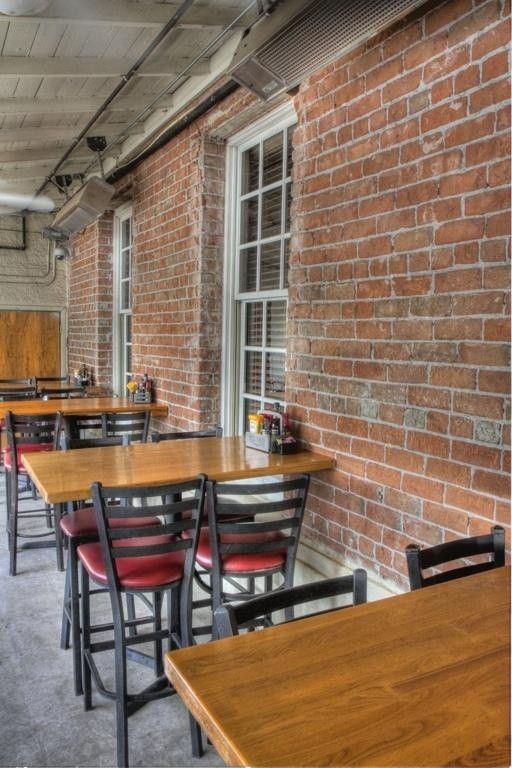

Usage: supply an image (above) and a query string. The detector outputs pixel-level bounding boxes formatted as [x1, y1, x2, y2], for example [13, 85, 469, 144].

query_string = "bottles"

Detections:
[271, 402, 290, 436]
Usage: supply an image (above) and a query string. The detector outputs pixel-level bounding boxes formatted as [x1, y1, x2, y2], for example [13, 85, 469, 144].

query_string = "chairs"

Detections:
[74, 473, 208, 767]
[101, 410, 151, 442]
[213, 568, 368, 636]
[2, 376, 110, 399]
[4, 409, 66, 576]
[180, 474, 311, 748]
[2, 395, 57, 452]
[57, 434, 162, 649]
[405, 526, 506, 587]
[151, 425, 257, 524]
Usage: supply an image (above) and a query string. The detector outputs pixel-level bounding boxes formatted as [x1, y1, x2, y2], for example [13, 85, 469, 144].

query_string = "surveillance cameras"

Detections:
[54, 248, 66, 261]
